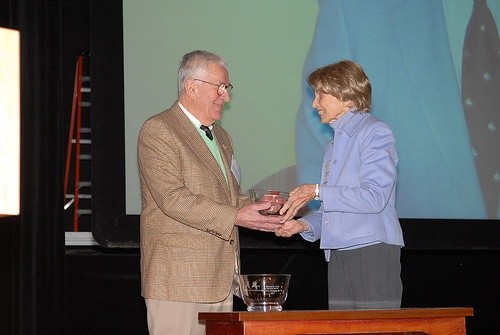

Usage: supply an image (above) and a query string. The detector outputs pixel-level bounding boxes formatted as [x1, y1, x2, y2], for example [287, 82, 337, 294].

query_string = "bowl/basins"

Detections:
[236, 274, 291, 311]
[249, 189, 290, 217]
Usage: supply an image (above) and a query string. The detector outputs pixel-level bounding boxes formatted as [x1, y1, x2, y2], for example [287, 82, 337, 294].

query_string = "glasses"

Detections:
[192, 78, 234, 97]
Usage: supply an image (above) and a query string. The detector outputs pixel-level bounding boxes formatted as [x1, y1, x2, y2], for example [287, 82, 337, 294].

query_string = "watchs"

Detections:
[313, 184, 319, 201]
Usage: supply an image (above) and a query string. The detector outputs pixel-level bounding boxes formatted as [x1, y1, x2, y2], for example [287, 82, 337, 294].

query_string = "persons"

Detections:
[274, 59, 405, 309]
[137, 50, 286, 335]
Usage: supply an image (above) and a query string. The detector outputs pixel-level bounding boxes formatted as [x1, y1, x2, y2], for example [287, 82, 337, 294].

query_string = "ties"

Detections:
[200, 124, 214, 141]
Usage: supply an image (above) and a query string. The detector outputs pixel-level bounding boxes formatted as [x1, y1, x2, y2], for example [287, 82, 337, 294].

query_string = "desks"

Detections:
[197, 307, 473, 335]
